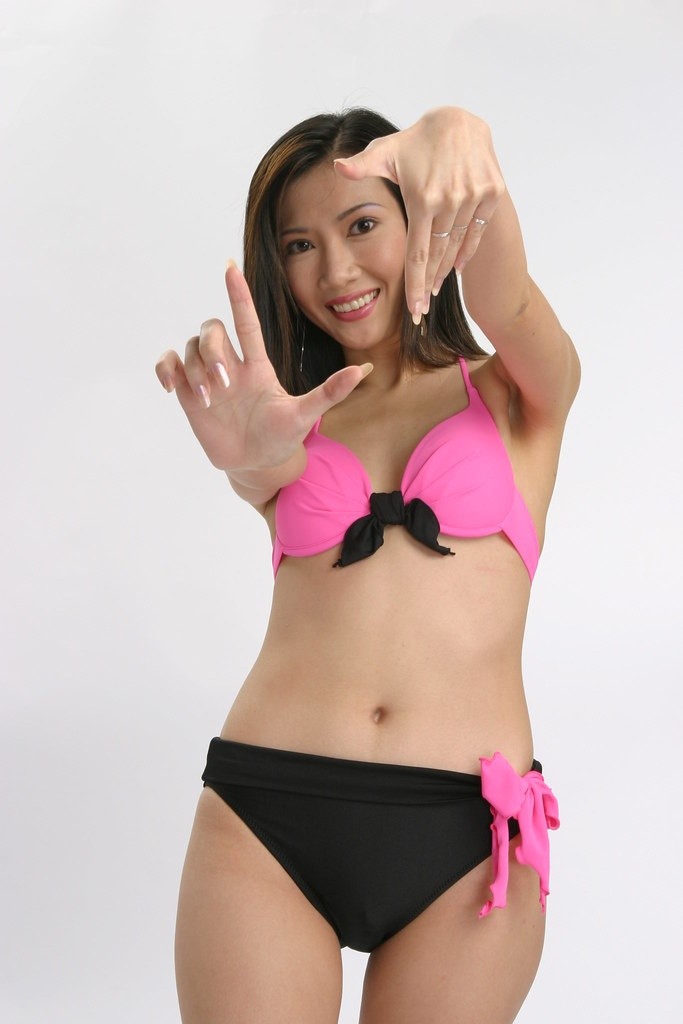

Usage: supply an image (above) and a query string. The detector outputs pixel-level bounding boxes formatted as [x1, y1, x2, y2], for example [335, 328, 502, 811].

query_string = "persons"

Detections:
[155, 105, 582, 1024]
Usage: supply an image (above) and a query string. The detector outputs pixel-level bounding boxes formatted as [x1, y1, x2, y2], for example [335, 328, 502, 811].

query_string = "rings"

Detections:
[472, 217, 489, 224]
[453, 226, 469, 230]
[431, 232, 450, 237]
